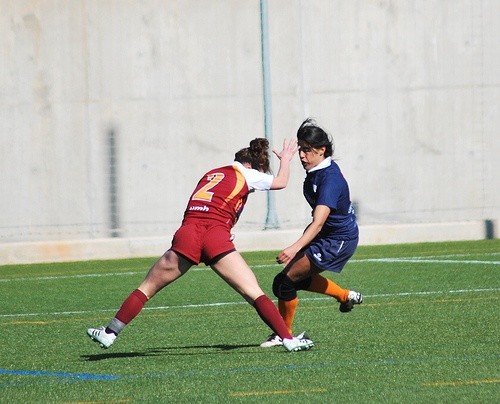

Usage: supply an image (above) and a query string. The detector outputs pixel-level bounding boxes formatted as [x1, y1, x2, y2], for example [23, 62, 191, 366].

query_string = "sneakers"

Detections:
[339, 290, 363, 313]
[260, 332, 283, 348]
[282, 331, 314, 352]
[86, 326, 116, 349]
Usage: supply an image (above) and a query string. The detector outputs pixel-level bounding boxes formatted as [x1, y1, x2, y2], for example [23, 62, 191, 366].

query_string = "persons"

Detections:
[87, 134, 316, 352]
[259, 117, 363, 346]
